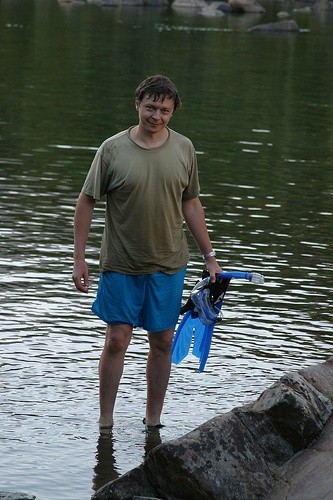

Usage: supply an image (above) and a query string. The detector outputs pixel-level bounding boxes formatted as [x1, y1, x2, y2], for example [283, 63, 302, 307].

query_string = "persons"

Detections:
[71, 76, 222, 425]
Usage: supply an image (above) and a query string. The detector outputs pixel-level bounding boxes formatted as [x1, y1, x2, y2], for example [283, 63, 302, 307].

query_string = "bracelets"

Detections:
[201, 251, 216, 260]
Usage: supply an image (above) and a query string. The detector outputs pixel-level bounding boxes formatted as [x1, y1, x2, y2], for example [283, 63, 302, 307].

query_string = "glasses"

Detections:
[191, 277, 222, 327]
